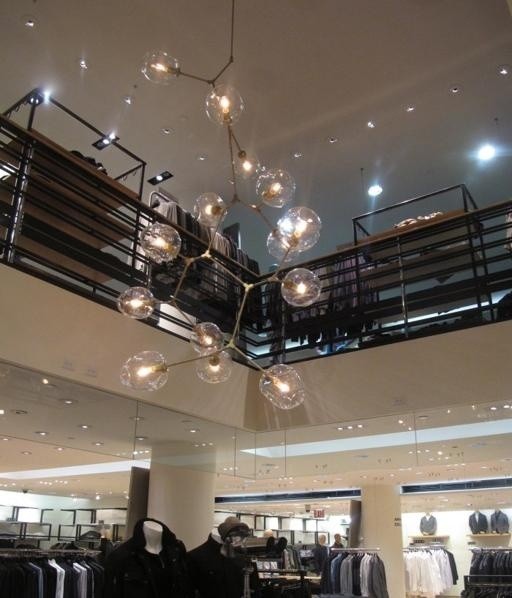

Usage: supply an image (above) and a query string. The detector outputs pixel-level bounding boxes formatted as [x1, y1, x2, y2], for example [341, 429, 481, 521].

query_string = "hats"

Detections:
[216, 516, 249, 540]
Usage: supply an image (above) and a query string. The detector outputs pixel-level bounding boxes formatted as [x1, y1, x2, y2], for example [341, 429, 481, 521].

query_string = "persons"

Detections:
[103, 517, 186, 598]
[330, 533, 344, 548]
[264, 529, 273, 538]
[419, 512, 437, 535]
[313, 535, 328, 574]
[491, 508, 509, 533]
[469, 511, 487, 534]
[186, 528, 260, 598]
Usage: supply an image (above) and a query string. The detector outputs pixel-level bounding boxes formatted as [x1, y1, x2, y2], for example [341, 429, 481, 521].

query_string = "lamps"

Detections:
[116, 0, 323, 408]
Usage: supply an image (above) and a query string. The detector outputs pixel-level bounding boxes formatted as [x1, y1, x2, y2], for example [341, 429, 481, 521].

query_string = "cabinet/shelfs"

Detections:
[0, 87, 147, 294]
[350, 183, 494, 348]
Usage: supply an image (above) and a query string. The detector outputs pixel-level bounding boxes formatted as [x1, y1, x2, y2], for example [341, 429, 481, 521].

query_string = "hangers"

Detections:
[0, 540, 97, 572]
[324, 548, 381, 568]
[401, 545, 446, 557]
